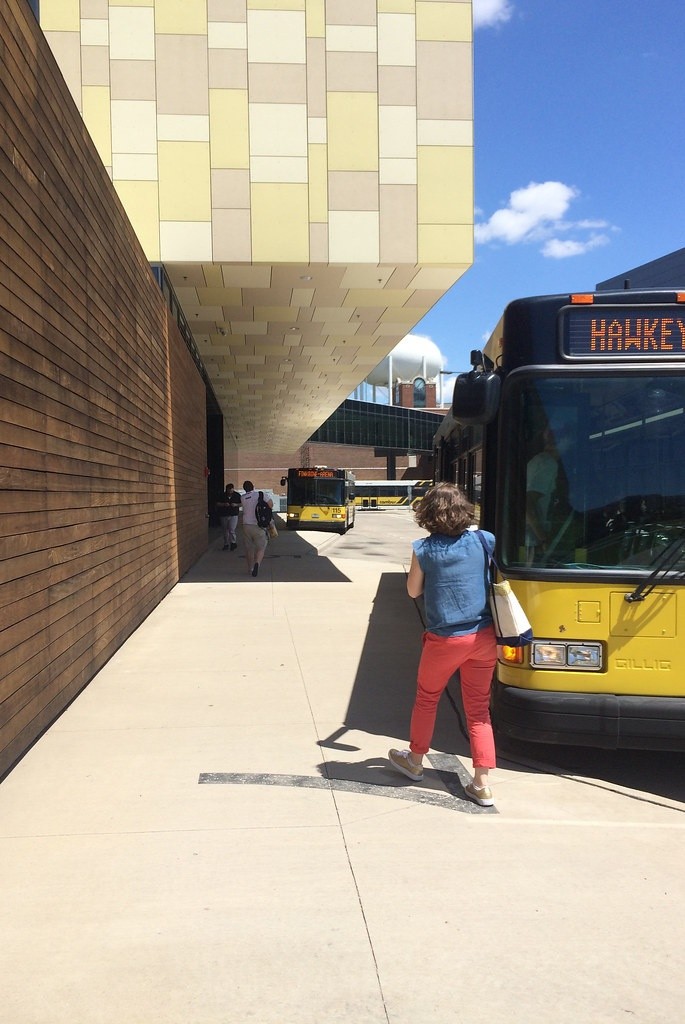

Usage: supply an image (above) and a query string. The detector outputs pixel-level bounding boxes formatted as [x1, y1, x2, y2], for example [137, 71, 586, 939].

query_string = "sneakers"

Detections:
[388, 749, 423, 781]
[465, 783, 494, 806]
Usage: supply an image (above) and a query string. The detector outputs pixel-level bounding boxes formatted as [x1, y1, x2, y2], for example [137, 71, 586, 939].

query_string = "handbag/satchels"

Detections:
[475, 530, 534, 647]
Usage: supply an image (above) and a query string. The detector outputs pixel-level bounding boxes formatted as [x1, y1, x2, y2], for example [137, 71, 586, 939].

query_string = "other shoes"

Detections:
[230, 543, 237, 551]
[248, 569, 253, 576]
[252, 562, 259, 577]
[222, 545, 229, 550]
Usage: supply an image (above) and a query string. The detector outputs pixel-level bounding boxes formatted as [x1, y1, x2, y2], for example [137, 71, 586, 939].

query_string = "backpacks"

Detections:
[255, 491, 272, 528]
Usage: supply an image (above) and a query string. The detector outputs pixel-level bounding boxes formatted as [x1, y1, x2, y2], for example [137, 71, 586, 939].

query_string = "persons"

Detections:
[524, 422, 570, 564]
[215, 483, 241, 551]
[324, 491, 338, 504]
[241, 481, 273, 577]
[387, 480, 498, 807]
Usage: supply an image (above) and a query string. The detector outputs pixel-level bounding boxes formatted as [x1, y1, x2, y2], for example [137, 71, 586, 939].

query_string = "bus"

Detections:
[281, 465, 355, 534]
[429, 287, 685, 757]
[345, 480, 433, 513]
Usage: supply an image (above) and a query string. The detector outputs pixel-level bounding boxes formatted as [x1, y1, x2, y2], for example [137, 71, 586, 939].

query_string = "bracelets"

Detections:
[540, 539, 548, 545]
